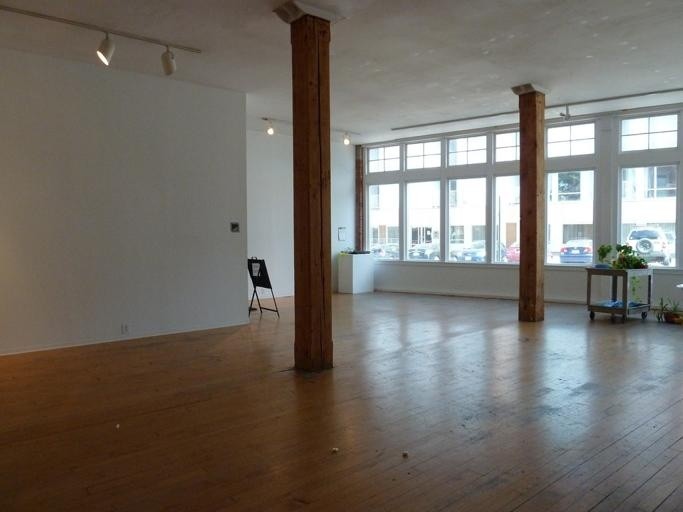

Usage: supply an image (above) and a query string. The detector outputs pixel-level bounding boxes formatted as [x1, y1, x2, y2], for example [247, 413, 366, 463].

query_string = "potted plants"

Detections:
[653, 297, 683, 324]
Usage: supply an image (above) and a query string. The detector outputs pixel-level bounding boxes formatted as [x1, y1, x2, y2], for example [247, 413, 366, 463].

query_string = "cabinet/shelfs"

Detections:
[587, 268, 654, 322]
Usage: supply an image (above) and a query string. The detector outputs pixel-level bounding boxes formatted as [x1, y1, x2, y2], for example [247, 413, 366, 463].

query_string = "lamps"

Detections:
[95, 30, 177, 75]
